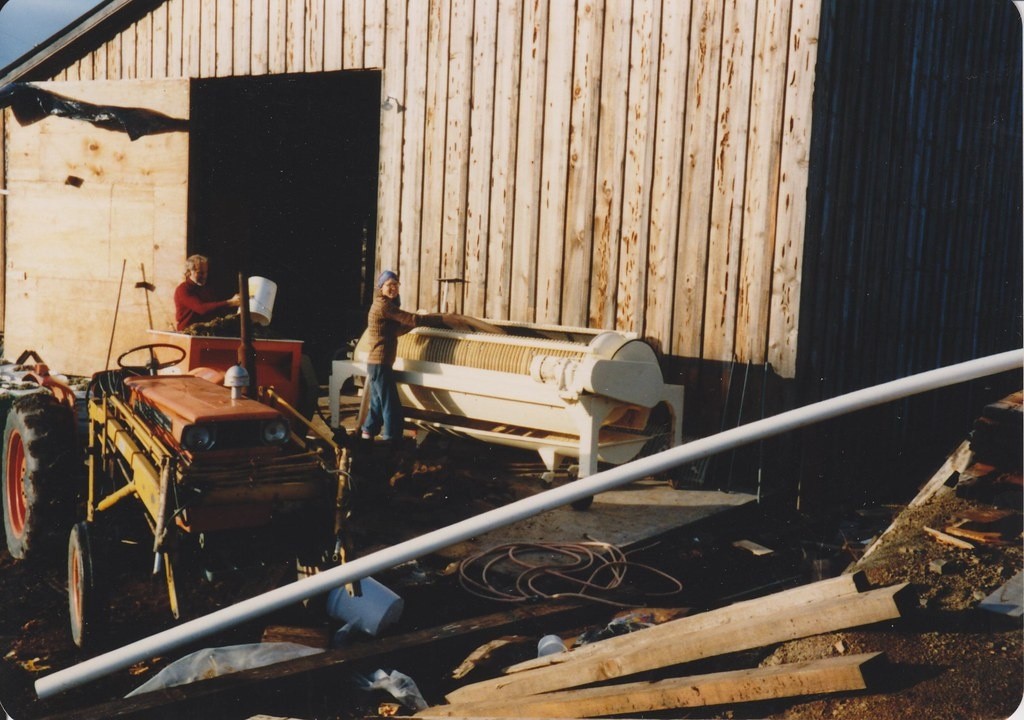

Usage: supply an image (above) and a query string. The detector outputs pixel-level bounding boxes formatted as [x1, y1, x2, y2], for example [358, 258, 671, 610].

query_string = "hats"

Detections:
[378, 271, 398, 289]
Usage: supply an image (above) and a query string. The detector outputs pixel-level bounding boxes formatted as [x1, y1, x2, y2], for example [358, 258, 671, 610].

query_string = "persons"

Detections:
[172, 254, 242, 333]
[360, 271, 459, 440]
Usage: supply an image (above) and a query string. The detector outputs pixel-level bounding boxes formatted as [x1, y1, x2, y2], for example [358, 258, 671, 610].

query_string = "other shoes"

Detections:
[362, 430, 370, 439]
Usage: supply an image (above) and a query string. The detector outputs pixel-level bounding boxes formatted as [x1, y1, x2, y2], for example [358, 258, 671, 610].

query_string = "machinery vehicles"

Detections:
[1, 273, 375, 650]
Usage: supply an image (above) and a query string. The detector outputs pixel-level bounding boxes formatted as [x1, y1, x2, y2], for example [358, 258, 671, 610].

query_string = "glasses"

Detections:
[383, 282, 402, 287]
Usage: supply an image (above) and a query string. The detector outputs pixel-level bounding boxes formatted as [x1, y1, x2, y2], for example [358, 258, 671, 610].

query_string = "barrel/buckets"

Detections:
[537, 634, 567, 657]
[236, 276, 278, 326]
[326, 575, 404, 635]
